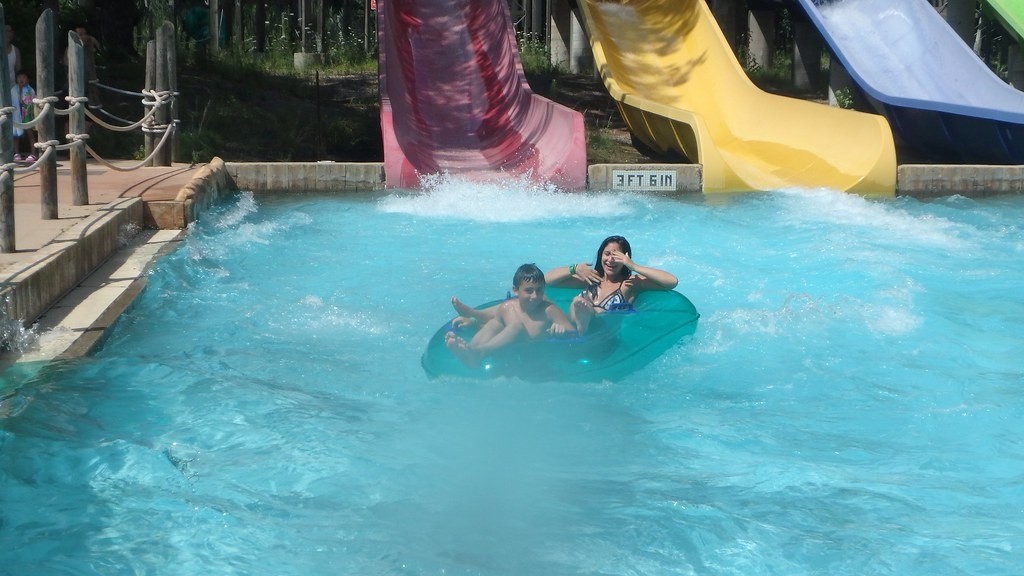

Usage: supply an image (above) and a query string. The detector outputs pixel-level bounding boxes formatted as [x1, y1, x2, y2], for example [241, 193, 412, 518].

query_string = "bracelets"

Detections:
[444, 263, 576, 370]
[569, 263, 579, 275]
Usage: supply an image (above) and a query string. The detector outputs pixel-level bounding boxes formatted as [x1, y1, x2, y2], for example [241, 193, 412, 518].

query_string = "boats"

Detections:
[421, 271, 700, 384]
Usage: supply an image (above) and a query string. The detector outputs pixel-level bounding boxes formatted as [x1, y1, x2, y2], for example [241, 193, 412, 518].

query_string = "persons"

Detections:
[4, 25, 38, 163]
[63, 25, 94, 159]
[450, 235, 679, 337]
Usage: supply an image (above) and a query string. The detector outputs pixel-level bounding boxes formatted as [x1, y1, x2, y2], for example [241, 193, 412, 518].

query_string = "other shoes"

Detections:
[14, 153, 22, 160]
[26, 155, 37, 161]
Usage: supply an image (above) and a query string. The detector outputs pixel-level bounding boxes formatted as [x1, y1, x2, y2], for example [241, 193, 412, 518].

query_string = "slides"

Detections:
[804, 0, 1024, 137]
[984, 1, 1024, 44]
[375, 1, 588, 197]
[573, 0, 898, 202]
